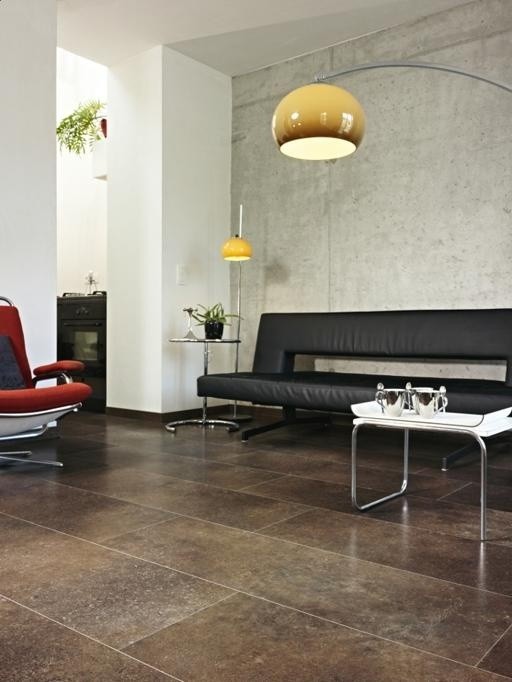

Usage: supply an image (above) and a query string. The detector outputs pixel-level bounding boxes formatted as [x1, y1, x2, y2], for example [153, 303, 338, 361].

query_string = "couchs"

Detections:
[196, 307, 512, 472]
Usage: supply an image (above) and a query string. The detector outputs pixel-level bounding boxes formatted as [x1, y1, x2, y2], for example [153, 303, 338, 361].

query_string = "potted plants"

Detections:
[192, 302, 245, 340]
[56, 97, 107, 155]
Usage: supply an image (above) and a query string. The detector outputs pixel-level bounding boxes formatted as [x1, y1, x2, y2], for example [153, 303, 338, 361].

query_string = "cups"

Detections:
[375, 389, 405, 417]
[405, 387, 448, 417]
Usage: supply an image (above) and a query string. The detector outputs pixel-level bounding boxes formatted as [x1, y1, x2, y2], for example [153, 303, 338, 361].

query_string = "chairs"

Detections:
[0, 295, 93, 468]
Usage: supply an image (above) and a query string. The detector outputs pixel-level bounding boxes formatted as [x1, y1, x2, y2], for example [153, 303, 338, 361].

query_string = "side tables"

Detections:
[165, 338, 241, 433]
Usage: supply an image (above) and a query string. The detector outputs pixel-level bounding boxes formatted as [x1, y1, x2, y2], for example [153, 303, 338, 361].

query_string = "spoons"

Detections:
[405, 382, 412, 411]
[377, 383, 386, 414]
[439, 386, 446, 414]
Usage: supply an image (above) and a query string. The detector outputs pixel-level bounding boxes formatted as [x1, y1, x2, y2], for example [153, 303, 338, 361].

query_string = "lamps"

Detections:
[218, 203, 253, 424]
[270, 62, 512, 161]
